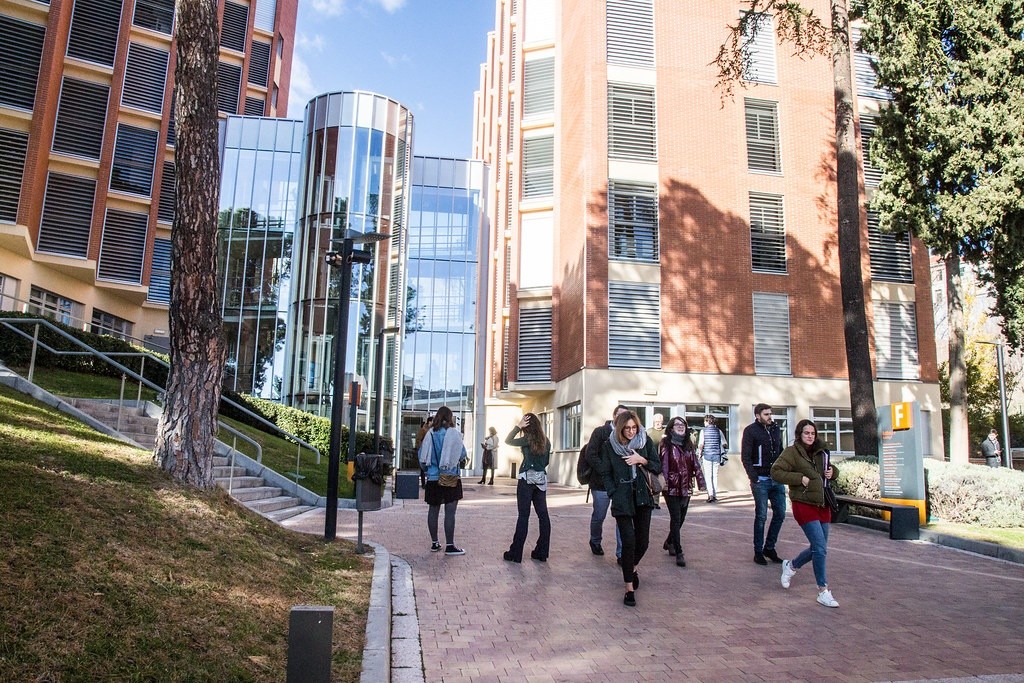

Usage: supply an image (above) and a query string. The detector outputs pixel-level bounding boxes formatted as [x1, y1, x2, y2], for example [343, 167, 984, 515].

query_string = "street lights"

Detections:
[323, 232, 396, 544]
[374, 325, 400, 454]
[975, 338, 1013, 468]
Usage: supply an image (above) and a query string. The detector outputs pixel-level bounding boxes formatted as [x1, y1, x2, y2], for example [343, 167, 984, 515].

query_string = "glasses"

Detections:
[623, 425, 637, 431]
[673, 423, 684, 428]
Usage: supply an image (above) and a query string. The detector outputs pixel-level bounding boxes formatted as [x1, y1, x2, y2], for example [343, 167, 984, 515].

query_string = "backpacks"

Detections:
[577, 426, 608, 485]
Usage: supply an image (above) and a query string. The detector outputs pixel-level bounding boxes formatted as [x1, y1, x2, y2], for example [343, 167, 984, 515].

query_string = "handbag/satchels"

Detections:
[526, 469, 545, 485]
[647, 470, 666, 493]
[824, 487, 839, 512]
[438, 473, 458, 488]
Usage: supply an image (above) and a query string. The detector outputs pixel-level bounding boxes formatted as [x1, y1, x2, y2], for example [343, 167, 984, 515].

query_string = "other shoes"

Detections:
[754, 555, 767, 565]
[707, 495, 716, 502]
[531, 550, 546, 562]
[503, 551, 521, 562]
[763, 549, 782, 563]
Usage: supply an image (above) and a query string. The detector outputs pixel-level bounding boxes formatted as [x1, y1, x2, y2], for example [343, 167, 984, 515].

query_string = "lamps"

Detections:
[153, 327, 165, 334]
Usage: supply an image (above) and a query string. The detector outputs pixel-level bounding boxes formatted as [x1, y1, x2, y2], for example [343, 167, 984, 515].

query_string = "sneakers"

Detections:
[431, 541, 442, 551]
[817, 589, 839, 608]
[589, 540, 604, 555]
[617, 558, 621, 564]
[624, 591, 636, 606]
[632, 569, 639, 589]
[445, 544, 465, 555]
[780, 560, 796, 588]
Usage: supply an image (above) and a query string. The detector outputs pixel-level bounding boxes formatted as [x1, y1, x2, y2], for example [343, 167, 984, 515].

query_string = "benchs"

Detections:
[395, 472, 420, 499]
[829, 495, 920, 541]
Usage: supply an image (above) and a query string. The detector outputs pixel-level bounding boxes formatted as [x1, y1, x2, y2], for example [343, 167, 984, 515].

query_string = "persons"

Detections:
[585, 405, 622, 564]
[416, 416, 434, 489]
[646, 414, 667, 509]
[418, 406, 466, 555]
[741, 403, 786, 565]
[698, 414, 727, 503]
[770, 420, 839, 607]
[503, 413, 551, 562]
[981, 428, 1001, 467]
[659, 417, 707, 567]
[477, 427, 499, 485]
[601, 411, 662, 606]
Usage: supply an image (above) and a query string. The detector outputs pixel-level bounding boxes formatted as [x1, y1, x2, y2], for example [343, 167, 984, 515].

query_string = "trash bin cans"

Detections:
[355, 455, 382, 511]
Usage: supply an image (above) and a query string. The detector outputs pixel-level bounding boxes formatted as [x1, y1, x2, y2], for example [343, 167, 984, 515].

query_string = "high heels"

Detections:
[488, 481, 493, 485]
[663, 540, 676, 555]
[676, 553, 685, 566]
[478, 479, 486, 484]
